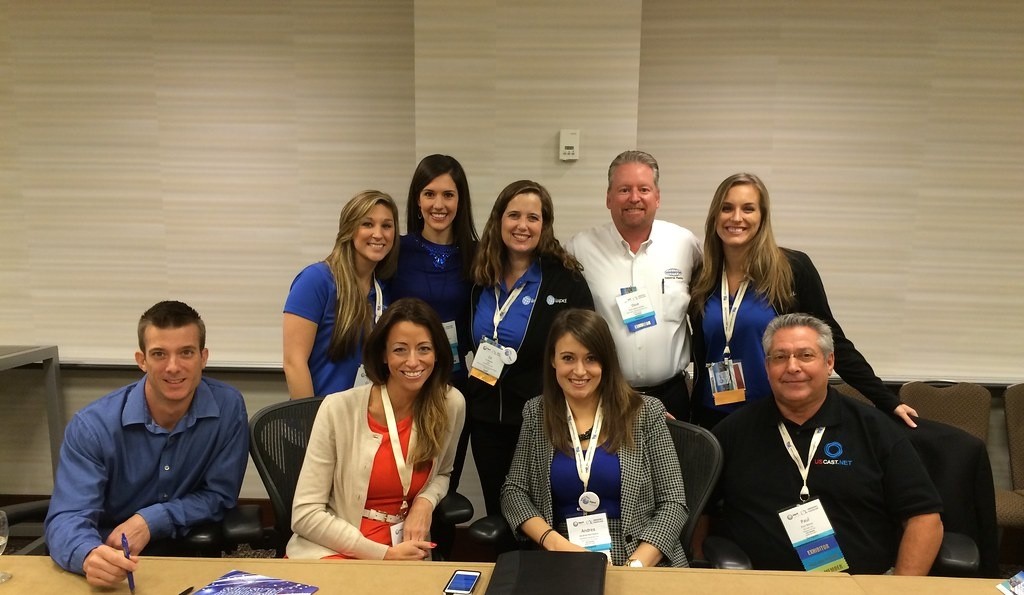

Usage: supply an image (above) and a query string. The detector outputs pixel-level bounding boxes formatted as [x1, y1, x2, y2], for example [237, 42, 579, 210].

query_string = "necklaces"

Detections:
[373, 266, 385, 324]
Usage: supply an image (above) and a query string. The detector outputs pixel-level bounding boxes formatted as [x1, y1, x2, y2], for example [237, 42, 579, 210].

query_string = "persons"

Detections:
[703, 314, 945, 577]
[282, 190, 400, 481]
[44, 300, 248, 589]
[689, 173, 919, 429]
[403, 154, 485, 493]
[470, 180, 594, 549]
[501, 309, 690, 568]
[566, 151, 703, 425]
[281, 297, 465, 561]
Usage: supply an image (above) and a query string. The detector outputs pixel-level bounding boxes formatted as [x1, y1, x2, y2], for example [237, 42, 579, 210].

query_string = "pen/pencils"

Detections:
[121, 533, 135, 595]
[662, 278, 664, 294]
[179, 586, 194, 595]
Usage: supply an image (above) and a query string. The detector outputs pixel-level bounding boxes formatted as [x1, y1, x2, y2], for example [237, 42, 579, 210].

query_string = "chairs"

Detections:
[1003, 383, 1024, 498]
[247, 396, 475, 564]
[465, 419, 723, 576]
[710, 415, 995, 578]
[900, 380, 1024, 524]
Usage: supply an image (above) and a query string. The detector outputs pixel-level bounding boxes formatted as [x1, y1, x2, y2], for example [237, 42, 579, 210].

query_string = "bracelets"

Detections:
[538, 529, 554, 546]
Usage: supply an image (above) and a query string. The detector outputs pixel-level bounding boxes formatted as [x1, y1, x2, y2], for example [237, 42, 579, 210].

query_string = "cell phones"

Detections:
[443, 570, 481, 595]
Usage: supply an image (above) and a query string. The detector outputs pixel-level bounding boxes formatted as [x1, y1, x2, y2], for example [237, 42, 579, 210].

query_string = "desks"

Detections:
[0, 555, 1024, 595]
[0, 340, 66, 556]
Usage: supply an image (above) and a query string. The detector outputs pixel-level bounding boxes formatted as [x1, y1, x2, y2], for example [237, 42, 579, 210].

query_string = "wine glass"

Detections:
[0, 510, 12, 585]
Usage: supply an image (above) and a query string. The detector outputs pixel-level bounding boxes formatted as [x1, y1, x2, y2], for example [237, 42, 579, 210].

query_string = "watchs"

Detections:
[625, 558, 644, 567]
